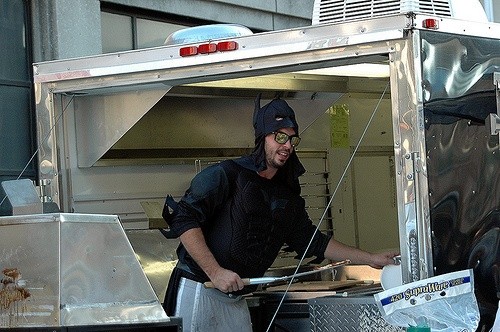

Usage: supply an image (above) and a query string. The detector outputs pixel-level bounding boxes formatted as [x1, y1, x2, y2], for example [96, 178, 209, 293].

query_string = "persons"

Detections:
[157, 97, 401, 332]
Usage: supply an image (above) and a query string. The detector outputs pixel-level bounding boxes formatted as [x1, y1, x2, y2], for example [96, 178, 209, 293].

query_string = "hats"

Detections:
[253, 92, 306, 176]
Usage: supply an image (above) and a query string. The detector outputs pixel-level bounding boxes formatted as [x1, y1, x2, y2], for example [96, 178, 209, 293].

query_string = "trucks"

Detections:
[0, 0, 500, 330]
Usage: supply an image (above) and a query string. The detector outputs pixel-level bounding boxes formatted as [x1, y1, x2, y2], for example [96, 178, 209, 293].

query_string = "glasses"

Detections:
[270, 128, 300, 148]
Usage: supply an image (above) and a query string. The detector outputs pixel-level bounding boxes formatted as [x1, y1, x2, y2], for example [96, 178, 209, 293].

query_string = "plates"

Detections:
[380, 255, 402, 291]
